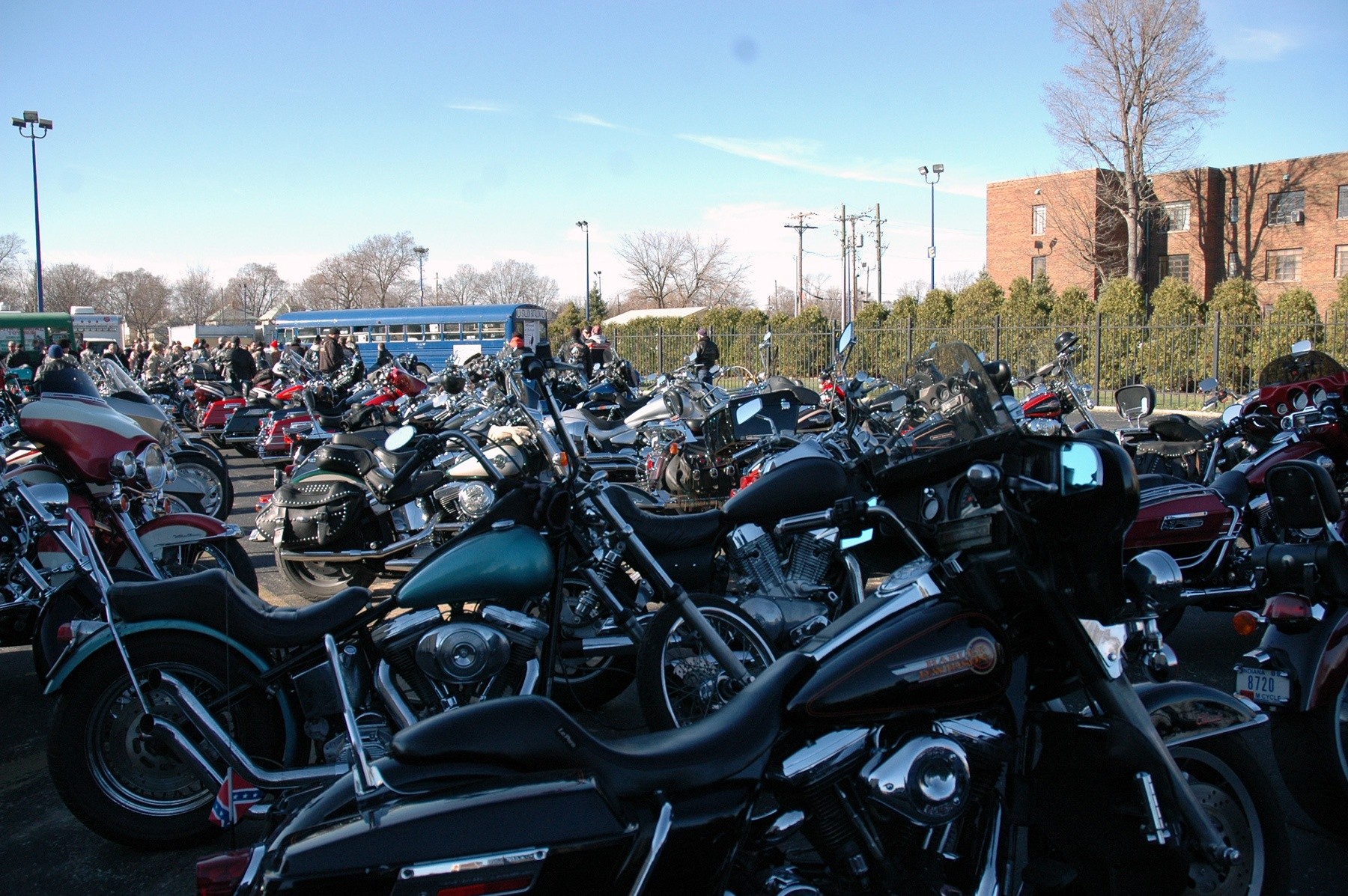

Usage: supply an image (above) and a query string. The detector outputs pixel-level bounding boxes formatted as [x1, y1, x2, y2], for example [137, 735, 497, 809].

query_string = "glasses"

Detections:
[584, 332, 591, 334]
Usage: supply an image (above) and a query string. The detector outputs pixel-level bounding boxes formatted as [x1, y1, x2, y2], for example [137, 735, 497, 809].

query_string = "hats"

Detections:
[592, 325, 602, 334]
[48, 344, 63, 359]
[571, 327, 580, 337]
[231, 335, 240, 343]
[698, 329, 707, 336]
[585, 326, 592, 331]
[331, 327, 340, 334]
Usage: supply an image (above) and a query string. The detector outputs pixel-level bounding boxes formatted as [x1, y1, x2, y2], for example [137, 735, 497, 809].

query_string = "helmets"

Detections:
[441, 375, 466, 394]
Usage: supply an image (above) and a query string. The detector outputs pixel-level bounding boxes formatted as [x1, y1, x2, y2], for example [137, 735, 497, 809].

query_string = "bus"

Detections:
[0, 311, 78, 355]
[70, 307, 123, 354]
[269, 304, 551, 367]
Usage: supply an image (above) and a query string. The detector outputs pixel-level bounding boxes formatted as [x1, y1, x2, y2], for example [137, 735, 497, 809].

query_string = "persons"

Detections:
[510, 330, 536, 358]
[377, 342, 394, 361]
[556, 325, 607, 379]
[6, 327, 361, 397]
[689, 328, 720, 385]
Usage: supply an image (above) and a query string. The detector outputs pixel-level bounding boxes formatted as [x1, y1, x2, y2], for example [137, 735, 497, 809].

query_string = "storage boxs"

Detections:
[189, 360, 218, 380]
[263, 412, 321, 450]
[220, 405, 276, 436]
[697, 376, 801, 458]
[202, 398, 246, 425]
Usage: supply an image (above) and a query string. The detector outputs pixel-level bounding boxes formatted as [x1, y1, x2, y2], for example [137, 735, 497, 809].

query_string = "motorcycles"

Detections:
[0, 341, 1348, 896]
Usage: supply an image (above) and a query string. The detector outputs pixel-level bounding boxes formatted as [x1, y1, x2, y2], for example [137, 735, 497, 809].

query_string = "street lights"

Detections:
[12, 110, 53, 310]
[918, 163, 945, 293]
[576, 221, 589, 322]
[413, 246, 429, 307]
[593, 271, 602, 297]
[239, 284, 247, 325]
